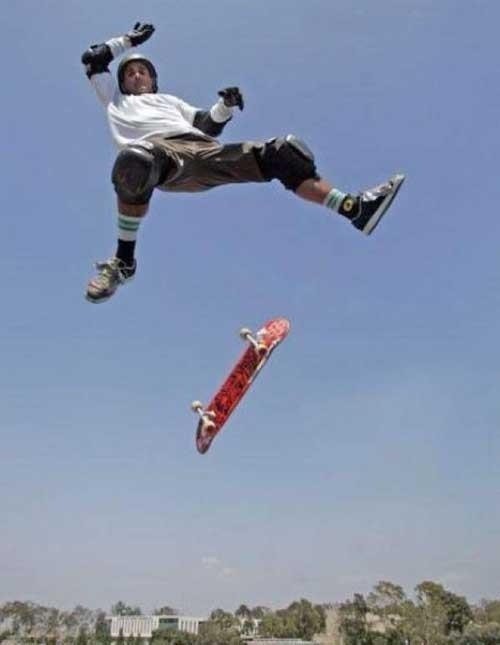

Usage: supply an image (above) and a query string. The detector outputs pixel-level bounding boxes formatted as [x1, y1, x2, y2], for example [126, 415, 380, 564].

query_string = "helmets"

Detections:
[118, 53, 157, 94]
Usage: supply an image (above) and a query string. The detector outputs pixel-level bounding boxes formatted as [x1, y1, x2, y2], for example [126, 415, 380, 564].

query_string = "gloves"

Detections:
[219, 87, 243, 110]
[125, 22, 155, 46]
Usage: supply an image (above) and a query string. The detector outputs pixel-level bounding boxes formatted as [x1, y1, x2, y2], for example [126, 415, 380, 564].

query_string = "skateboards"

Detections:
[190, 318, 291, 454]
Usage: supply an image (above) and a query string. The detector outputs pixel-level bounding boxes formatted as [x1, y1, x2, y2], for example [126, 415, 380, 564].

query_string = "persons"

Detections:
[81, 20, 406, 304]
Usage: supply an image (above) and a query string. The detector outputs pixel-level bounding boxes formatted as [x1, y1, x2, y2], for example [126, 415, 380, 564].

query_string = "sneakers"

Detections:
[86, 256, 136, 303]
[351, 174, 405, 235]
